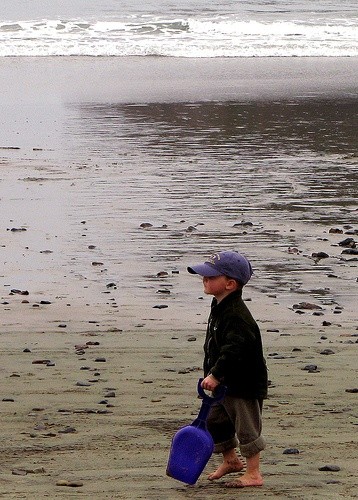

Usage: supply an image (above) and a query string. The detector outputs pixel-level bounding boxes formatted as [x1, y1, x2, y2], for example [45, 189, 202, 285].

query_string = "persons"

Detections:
[188, 250, 270, 488]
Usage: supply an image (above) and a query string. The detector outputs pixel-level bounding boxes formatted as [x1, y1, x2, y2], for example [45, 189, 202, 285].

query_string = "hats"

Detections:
[188, 251, 253, 284]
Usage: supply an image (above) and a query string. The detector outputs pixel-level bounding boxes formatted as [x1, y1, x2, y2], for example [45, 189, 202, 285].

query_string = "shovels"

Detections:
[166, 378, 227, 486]
